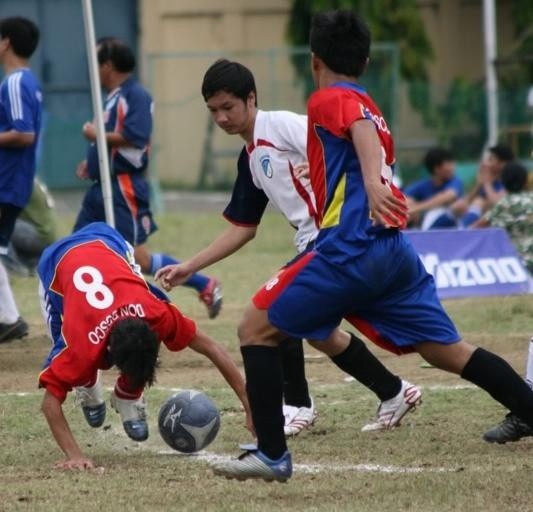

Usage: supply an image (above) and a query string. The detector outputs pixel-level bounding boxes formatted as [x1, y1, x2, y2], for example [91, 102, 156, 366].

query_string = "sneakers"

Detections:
[110, 392, 149, 441]
[76, 370, 106, 427]
[206, 448, 292, 483]
[200, 277, 223, 319]
[283, 396, 314, 435]
[484, 412, 533, 443]
[362, 380, 421, 432]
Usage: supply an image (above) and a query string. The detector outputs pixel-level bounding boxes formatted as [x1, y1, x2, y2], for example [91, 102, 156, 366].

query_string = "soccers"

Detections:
[158, 390, 220, 452]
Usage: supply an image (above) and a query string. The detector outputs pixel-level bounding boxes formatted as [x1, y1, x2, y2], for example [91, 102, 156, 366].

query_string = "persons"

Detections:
[0, 17, 45, 343]
[70, 35, 224, 319]
[402, 143, 533, 277]
[35, 222, 256, 472]
[201, 8, 533, 481]
[153, 59, 423, 438]
[7, 180, 57, 275]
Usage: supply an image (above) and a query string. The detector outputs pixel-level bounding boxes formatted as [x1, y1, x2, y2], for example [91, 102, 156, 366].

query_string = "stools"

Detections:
[505, 126, 533, 158]
